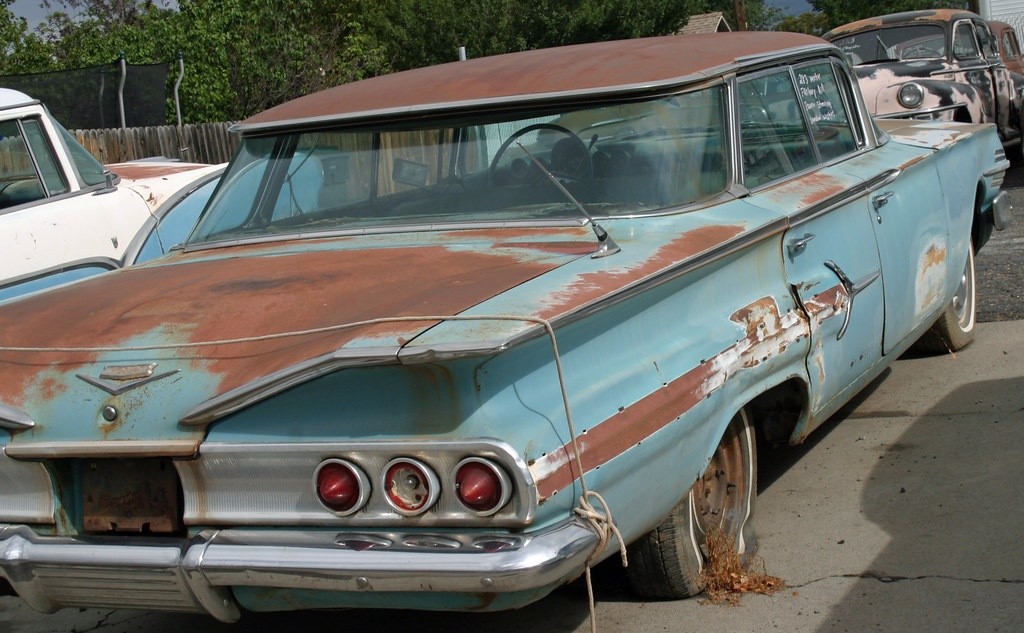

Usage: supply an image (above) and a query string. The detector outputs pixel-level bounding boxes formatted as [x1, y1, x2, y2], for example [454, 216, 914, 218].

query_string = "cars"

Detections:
[0, 33, 1009, 612]
[821, 9, 1024, 172]
[985, 20, 1024, 75]
[0, 88, 222, 283]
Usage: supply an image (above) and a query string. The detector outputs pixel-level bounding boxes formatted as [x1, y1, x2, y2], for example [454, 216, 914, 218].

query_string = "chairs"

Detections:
[388, 132, 844, 218]
